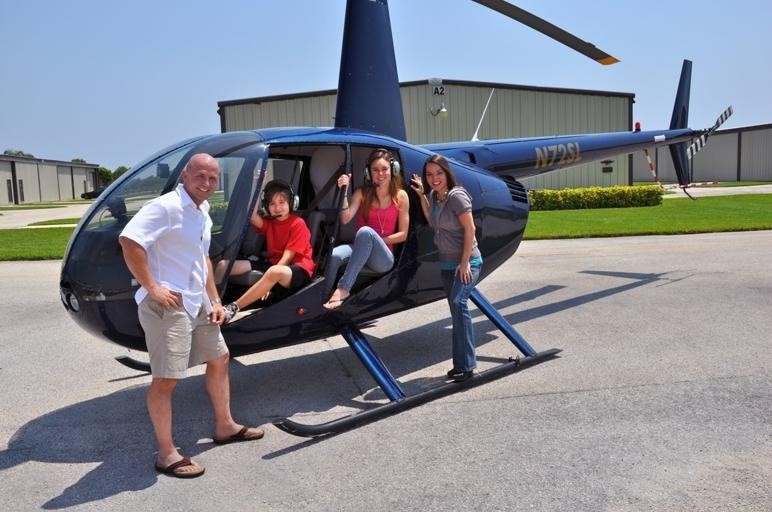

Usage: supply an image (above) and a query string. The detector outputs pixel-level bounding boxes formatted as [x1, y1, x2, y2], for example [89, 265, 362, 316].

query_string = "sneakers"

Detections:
[447, 368, 474, 382]
[221, 300, 239, 324]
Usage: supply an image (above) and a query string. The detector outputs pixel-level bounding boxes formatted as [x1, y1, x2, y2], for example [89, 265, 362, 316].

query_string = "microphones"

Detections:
[267, 215, 281, 219]
[370, 181, 381, 188]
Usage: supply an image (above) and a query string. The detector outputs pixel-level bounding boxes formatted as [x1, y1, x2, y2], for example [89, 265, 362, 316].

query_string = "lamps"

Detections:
[429, 101, 448, 118]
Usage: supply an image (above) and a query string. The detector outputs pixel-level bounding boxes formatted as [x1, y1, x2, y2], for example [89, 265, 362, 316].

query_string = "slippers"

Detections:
[154, 455, 206, 479]
[322, 295, 348, 311]
[212, 424, 267, 445]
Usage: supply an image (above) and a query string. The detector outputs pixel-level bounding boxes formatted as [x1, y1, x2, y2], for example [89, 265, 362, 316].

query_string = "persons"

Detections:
[117, 151, 266, 478]
[322, 147, 413, 313]
[211, 177, 317, 324]
[409, 154, 484, 383]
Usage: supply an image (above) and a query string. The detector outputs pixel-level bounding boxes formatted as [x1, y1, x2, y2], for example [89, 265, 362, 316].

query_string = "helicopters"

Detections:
[60, 0, 732, 438]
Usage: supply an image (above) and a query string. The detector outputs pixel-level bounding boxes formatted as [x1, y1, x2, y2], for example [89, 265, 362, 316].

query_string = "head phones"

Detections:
[366, 148, 400, 179]
[260, 179, 301, 214]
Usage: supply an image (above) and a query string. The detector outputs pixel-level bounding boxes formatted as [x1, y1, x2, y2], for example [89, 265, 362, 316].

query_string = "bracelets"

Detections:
[339, 194, 349, 212]
[210, 297, 222, 307]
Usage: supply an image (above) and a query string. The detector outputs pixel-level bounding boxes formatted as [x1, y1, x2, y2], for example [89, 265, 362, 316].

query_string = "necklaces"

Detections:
[373, 190, 393, 235]
[435, 188, 449, 204]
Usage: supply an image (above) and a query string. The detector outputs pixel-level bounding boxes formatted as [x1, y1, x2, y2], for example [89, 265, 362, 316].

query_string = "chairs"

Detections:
[229, 208, 327, 287]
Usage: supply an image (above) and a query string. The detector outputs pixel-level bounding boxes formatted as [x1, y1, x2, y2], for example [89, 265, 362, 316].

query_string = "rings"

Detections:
[463, 272, 467, 276]
